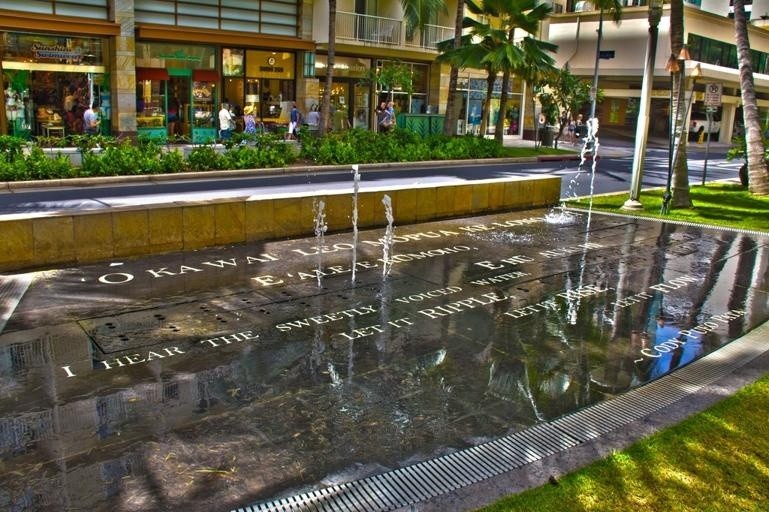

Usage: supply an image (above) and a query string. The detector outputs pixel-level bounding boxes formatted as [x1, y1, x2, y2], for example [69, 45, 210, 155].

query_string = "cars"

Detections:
[690, 112, 721, 134]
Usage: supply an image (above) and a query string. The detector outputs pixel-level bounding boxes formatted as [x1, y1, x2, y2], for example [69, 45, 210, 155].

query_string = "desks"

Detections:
[41, 124, 65, 138]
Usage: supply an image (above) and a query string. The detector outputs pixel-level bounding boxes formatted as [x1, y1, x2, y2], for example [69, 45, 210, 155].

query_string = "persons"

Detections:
[167, 93, 179, 136]
[217, 102, 320, 147]
[82, 99, 101, 136]
[539, 110, 583, 147]
[375, 101, 396, 133]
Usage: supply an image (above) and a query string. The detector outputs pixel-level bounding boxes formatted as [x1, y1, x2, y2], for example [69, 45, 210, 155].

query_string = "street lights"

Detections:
[661, 41, 701, 217]
